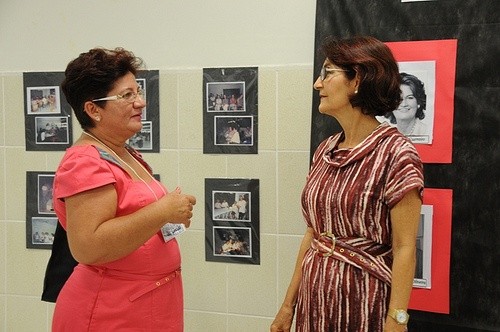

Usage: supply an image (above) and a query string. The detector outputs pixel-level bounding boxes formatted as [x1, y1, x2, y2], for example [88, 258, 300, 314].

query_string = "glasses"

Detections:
[320, 67, 350, 81]
[91, 89, 143, 102]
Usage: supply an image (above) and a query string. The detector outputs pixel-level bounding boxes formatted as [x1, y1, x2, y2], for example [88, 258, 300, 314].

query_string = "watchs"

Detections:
[387, 309, 410, 325]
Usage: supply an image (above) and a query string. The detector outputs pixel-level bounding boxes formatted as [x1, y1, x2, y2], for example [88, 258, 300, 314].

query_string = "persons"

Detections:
[52, 48, 197, 332]
[222, 239, 248, 255]
[44, 123, 66, 142]
[270, 34, 424, 332]
[210, 93, 239, 110]
[377, 73, 430, 142]
[224, 121, 244, 144]
[215, 196, 248, 219]
[35, 232, 55, 243]
[32, 94, 55, 112]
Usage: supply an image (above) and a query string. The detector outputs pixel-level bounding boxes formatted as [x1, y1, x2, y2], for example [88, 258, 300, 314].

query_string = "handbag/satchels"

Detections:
[40, 219, 79, 303]
